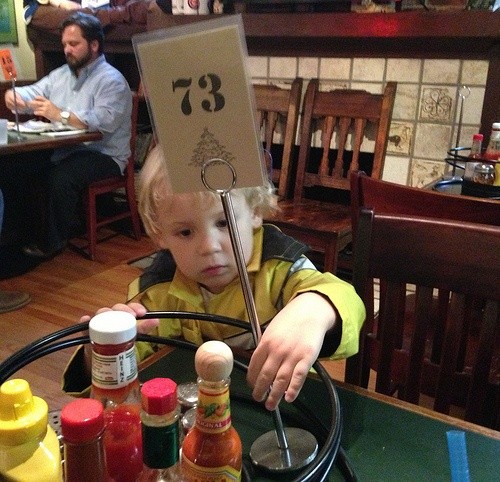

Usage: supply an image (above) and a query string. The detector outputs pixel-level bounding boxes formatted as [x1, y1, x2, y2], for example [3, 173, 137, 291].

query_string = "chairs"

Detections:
[346, 167, 500, 428]
[252, 77, 398, 275]
[80, 91, 141, 261]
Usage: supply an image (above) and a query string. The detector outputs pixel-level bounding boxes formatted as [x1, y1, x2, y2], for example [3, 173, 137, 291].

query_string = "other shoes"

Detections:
[0, 288, 32, 312]
[22, 238, 69, 258]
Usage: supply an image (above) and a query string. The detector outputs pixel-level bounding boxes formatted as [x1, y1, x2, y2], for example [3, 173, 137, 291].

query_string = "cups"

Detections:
[0, 119, 8, 145]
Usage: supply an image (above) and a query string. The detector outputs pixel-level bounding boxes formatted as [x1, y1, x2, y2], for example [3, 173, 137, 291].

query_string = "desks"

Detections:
[88, 336, 500, 482]
[0, 121, 103, 280]
[421, 168, 465, 195]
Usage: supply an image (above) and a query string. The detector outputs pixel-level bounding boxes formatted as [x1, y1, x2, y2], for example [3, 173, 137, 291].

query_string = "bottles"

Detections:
[0, 379, 64, 482]
[89, 311, 142, 482]
[60, 399, 110, 482]
[464, 134, 483, 181]
[483, 122, 500, 165]
[181, 340, 241, 482]
[473, 164, 496, 185]
[136, 379, 180, 482]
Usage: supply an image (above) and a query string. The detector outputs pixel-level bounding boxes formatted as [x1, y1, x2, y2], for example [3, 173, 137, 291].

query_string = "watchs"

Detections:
[60, 109, 71, 125]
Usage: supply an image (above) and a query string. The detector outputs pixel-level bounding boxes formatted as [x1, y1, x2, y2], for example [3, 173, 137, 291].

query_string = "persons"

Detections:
[60, 143, 366, 410]
[3, 11, 133, 259]
